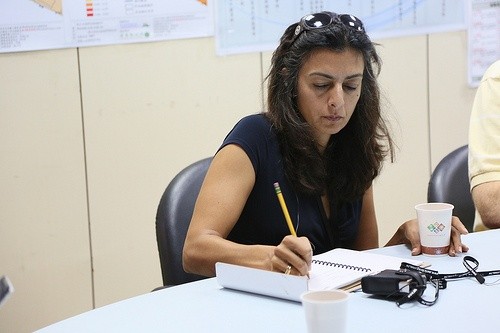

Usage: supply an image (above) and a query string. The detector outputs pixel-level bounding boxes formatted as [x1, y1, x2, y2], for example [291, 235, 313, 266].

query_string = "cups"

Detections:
[300, 290, 351, 333]
[415, 203, 454, 254]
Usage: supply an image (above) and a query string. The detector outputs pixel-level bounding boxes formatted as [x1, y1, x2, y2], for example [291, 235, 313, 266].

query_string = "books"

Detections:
[301, 248, 432, 294]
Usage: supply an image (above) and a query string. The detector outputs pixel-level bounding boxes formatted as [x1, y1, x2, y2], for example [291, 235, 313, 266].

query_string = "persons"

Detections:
[181, 11, 469, 277]
[467, 57, 500, 232]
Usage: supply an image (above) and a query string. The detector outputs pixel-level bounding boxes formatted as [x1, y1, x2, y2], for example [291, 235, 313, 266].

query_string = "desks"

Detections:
[28, 226, 500, 333]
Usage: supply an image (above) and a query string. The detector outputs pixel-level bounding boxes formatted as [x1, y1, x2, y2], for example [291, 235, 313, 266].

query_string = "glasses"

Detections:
[286, 13, 365, 50]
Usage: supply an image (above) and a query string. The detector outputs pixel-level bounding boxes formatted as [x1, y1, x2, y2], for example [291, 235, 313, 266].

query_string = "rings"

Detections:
[285, 265, 291, 275]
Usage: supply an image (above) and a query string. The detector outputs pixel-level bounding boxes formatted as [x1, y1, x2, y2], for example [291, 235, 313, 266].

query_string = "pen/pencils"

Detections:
[273, 181, 311, 281]
